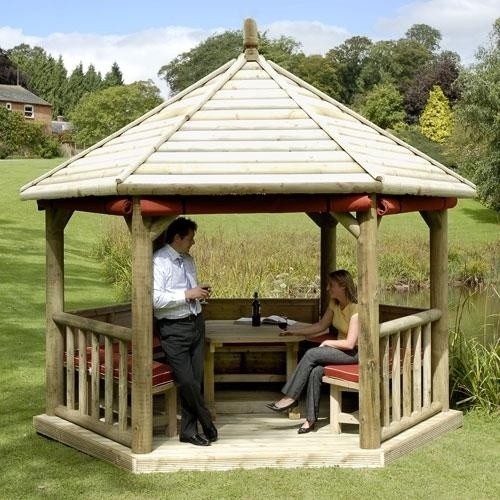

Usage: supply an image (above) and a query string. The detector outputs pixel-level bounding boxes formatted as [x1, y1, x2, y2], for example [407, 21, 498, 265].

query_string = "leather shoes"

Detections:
[180, 434, 210, 446]
[199, 408, 217, 442]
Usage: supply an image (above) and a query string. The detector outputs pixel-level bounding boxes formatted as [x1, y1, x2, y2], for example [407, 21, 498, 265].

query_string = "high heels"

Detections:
[264, 399, 298, 412]
[298, 419, 317, 433]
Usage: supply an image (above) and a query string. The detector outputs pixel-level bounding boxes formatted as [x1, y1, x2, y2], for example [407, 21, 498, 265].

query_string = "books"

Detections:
[233, 314, 297, 326]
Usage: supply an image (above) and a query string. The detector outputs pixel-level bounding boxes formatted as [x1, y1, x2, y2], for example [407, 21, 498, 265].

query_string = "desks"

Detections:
[202, 319, 329, 420]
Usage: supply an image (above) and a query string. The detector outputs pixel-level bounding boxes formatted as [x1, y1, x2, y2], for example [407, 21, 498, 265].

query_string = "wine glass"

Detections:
[278, 315, 288, 333]
[199, 280, 212, 305]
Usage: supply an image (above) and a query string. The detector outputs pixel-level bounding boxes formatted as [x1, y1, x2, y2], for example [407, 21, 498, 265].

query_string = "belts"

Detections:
[173, 315, 199, 321]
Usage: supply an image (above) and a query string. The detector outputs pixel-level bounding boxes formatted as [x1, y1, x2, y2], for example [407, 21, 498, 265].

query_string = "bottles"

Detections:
[251, 291, 261, 328]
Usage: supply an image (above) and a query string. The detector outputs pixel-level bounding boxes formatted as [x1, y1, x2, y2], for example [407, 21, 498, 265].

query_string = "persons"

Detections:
[152, 218, 218, 446]
[264, 269, 359, 434]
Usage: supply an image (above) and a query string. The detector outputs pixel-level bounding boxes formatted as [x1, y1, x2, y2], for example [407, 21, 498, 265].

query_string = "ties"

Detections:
[181, 256, 197, 316]
